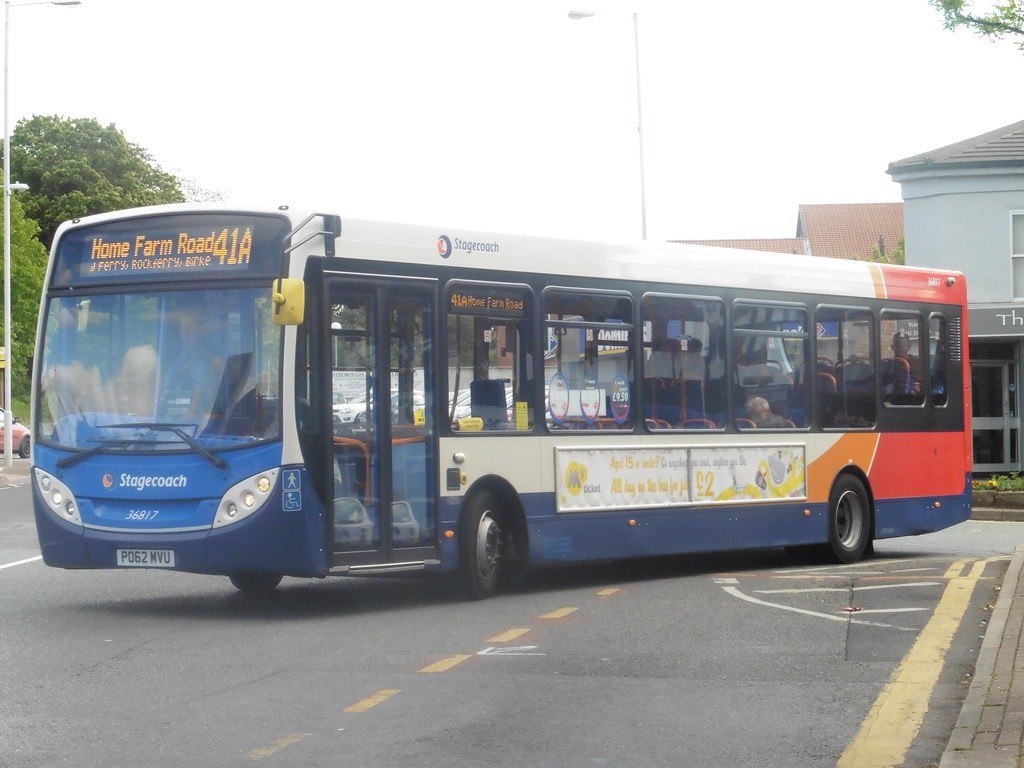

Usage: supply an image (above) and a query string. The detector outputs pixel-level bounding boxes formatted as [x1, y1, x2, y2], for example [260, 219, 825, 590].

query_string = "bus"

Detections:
[28, 203, 971, 600]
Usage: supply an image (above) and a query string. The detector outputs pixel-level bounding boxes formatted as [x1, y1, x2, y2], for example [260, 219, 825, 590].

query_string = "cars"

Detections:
[332, 385, 551, 429]
[2, 410, 31, 459]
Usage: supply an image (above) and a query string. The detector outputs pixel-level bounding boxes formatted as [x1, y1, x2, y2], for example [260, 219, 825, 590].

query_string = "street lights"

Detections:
[569, 8, 646, 240]
[3, 0, 84, 474]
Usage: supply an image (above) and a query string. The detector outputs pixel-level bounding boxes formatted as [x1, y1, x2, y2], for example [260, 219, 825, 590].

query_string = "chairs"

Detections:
[544, 350, 934, 434]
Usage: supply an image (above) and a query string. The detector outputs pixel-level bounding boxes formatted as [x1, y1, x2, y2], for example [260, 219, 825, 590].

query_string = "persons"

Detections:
[891, 332, 920, 392]
[746, 396, 786, 428]
[643, 337, 709, 389]
[164, 313, 236, 418]
